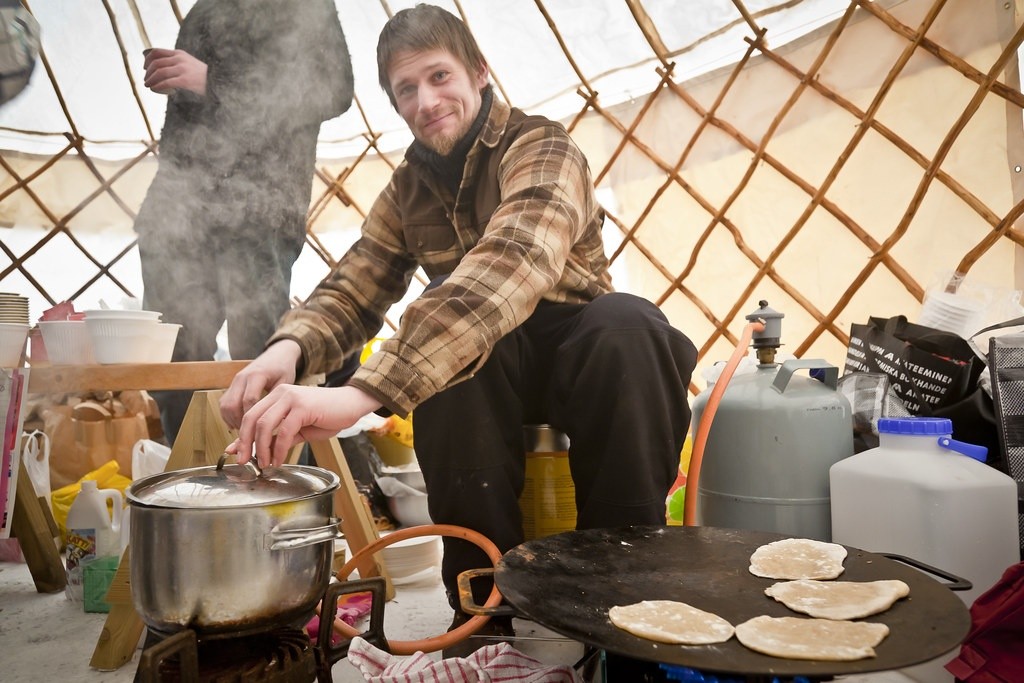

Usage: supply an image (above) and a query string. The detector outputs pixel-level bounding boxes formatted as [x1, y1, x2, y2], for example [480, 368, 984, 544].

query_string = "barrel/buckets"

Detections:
[827, 416, 1020, 683]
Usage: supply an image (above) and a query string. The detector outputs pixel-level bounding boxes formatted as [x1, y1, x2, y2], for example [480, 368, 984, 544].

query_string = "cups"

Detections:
[920, 292, 972, 335]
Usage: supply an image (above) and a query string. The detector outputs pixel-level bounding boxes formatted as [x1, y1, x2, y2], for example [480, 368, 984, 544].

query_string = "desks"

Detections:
[1, 359, 397, 671]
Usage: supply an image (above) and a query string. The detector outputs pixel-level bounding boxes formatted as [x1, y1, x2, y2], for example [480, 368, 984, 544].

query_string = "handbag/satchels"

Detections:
[843, 315, 985, 417]
[965, 315, 1024, 561]
[20, 401, 174, 542]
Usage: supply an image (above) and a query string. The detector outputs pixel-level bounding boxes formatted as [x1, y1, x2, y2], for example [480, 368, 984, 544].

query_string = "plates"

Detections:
[378, 533, 442, 586]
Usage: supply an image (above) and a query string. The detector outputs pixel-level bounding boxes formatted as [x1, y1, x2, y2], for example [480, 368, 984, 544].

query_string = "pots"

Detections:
[457, 525, 973, 673]
[122, 453, 342, 645]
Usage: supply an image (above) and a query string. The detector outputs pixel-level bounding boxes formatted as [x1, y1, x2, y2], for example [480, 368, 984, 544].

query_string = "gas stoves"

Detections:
[135, 574, 394, 683]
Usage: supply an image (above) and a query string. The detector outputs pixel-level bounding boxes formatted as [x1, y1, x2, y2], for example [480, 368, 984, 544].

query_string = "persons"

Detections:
[219, 4, 699, 683]
[133, 0, 354, 466]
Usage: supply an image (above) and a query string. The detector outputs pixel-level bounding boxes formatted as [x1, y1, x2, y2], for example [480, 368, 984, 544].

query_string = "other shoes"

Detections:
[442, 607, 516, 659]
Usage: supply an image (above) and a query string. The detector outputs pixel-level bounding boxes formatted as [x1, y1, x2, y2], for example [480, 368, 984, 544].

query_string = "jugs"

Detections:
[65, 482, 123, 600]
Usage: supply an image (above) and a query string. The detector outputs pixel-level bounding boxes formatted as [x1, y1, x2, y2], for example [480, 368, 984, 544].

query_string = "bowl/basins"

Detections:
[0, 293, 182, 367]
